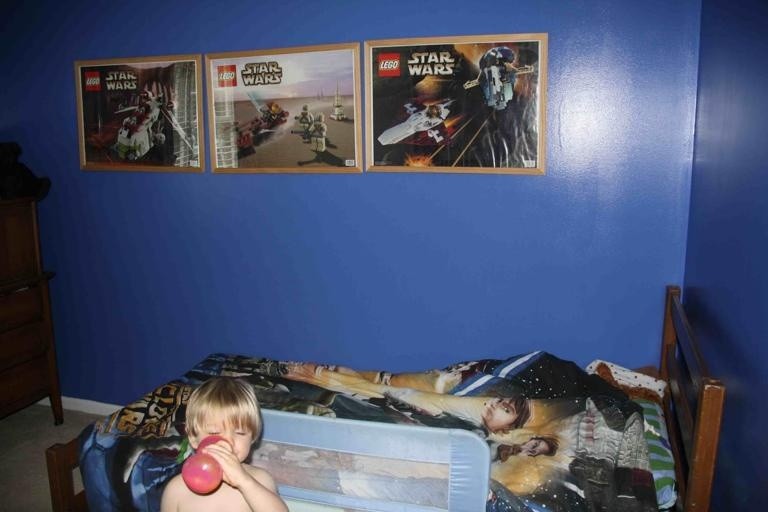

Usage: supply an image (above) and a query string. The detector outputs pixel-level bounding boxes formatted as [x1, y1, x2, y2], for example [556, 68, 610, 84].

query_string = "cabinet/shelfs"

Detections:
[2, 189, 63, 428]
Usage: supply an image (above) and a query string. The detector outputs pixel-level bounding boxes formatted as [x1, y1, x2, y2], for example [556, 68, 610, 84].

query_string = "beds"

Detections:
[82, 285, 730, 512]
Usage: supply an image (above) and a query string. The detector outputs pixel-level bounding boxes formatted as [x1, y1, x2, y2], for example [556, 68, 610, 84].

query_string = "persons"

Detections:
[363, 393, 558, 462]
[160, 379, 289, 512]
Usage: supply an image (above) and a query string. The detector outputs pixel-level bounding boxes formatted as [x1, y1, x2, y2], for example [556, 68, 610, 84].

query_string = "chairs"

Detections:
[45, 419, 88, 511]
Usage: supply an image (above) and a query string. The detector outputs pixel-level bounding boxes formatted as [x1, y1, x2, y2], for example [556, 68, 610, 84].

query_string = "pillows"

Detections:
[628, 397, 681, 511]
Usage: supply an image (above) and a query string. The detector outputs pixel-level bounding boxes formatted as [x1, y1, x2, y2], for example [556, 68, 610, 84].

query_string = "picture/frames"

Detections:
[75, 54, 202, 175]
[363, 35, 549, 175]
[204, 41, 361, 177]
